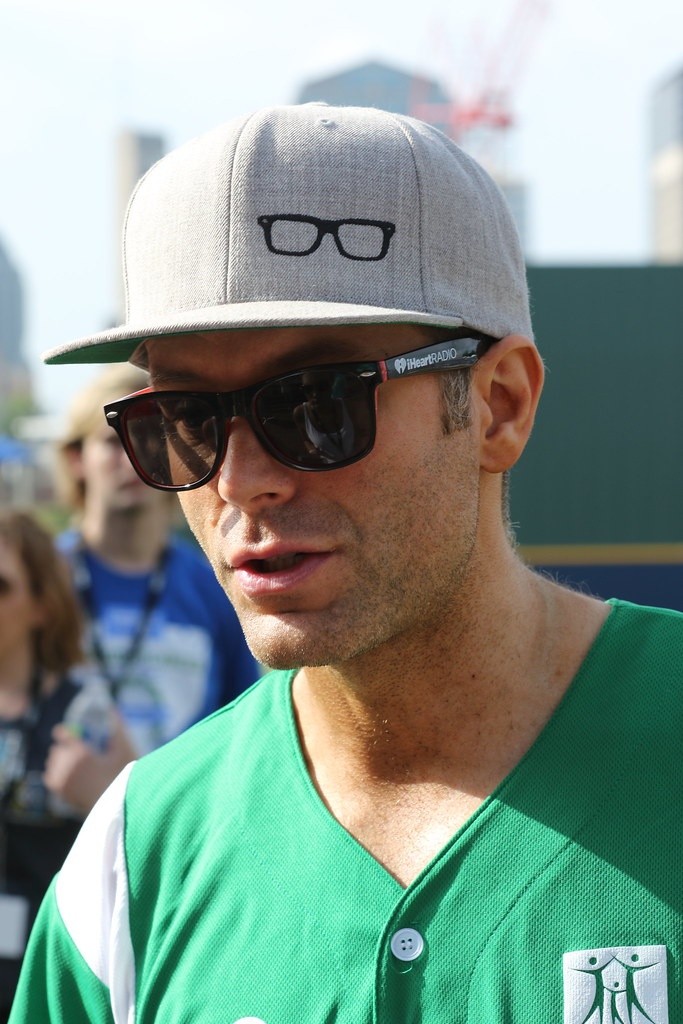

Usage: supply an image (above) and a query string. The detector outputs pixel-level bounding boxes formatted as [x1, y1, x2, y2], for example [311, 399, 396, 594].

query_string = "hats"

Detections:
[59, 360, 155, 447]
[39, 98, 542, 366]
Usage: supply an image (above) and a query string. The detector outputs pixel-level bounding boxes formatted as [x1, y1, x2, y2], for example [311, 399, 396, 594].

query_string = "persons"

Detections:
[0, 511, 142, 1024]
[6, 90, 683, 1024]
[36, 366, 264, 784]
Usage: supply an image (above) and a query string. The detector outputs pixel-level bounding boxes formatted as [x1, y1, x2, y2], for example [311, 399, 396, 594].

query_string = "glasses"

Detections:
[101, 334, 485, 491]
[0, 573, 32, 598]
